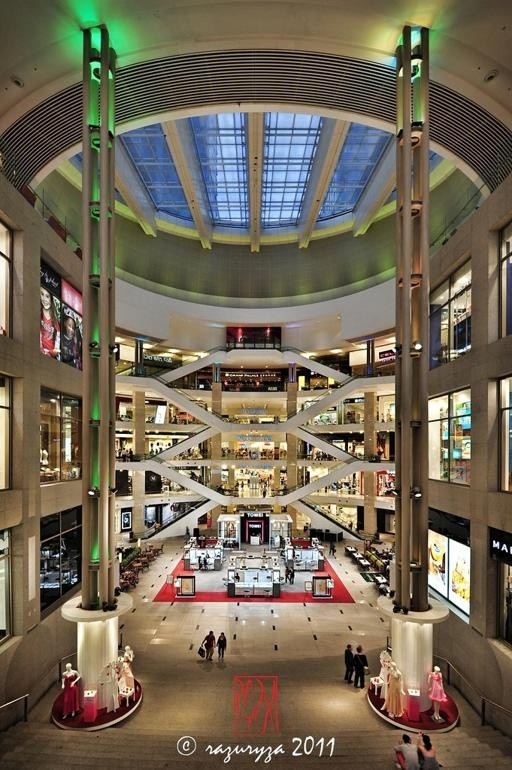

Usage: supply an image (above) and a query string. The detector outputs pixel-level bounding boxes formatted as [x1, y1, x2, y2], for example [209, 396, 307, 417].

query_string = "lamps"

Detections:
[409, 561, 417, 567]
[390, 488, 402, 496]
[410, 341, 423, 351]
[108, 343, 119, 355]
[109, 485, 120, 495]
[87, 487, 95, 497]
[411, 483, 424, 498]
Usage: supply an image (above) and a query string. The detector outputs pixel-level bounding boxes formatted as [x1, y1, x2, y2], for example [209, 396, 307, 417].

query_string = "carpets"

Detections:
[152, 538, 355, 603]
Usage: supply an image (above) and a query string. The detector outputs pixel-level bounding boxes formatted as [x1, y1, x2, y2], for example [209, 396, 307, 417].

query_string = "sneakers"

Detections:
[345, 677, 365, 688]
[206, 653, 224, 660]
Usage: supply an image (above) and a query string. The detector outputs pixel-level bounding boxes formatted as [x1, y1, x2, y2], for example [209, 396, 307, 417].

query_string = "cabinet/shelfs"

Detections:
[176, 536, 332, 598]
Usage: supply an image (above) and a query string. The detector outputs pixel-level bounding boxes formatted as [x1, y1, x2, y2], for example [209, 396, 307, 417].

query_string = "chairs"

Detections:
[117, 676, 135, 708]
[119, 543, 164, 592]
[369, 668, 385, 696]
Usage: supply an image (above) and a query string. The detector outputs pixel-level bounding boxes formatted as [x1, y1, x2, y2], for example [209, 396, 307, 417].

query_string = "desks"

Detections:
[344, 545, 390, 587]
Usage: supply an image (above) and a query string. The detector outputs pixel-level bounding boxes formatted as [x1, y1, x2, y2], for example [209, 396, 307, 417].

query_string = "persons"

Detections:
[60, 663, 81, 720]
[124, 514, 129, 526]
[290, 567, 295, 584]
[111, 404, 399, 497]
[343, 643, 355, 684]
[59, 311, 75, 366]
[395, 733, 419, 770]
[199, 554, 210, 571]
[123, 645, 136, 702]
[285, 566, 289, 584]
[329, 541, 338, 559]
[432, 667, 446, 721]
[40, 287, 63, 358]
[385, 662, 404, 719]
[217, 632, 228, 659]
[73, 323, 82, 370]
[417, 730, 440, 770]
[353, 645, 368, 689]
[202, 631, 216, 661]
[349, 520, 354, 530]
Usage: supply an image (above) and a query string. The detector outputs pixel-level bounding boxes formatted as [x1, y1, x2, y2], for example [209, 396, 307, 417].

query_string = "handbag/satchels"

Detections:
[365, 667, 370, 675]
[198, 647, 205, 657]
